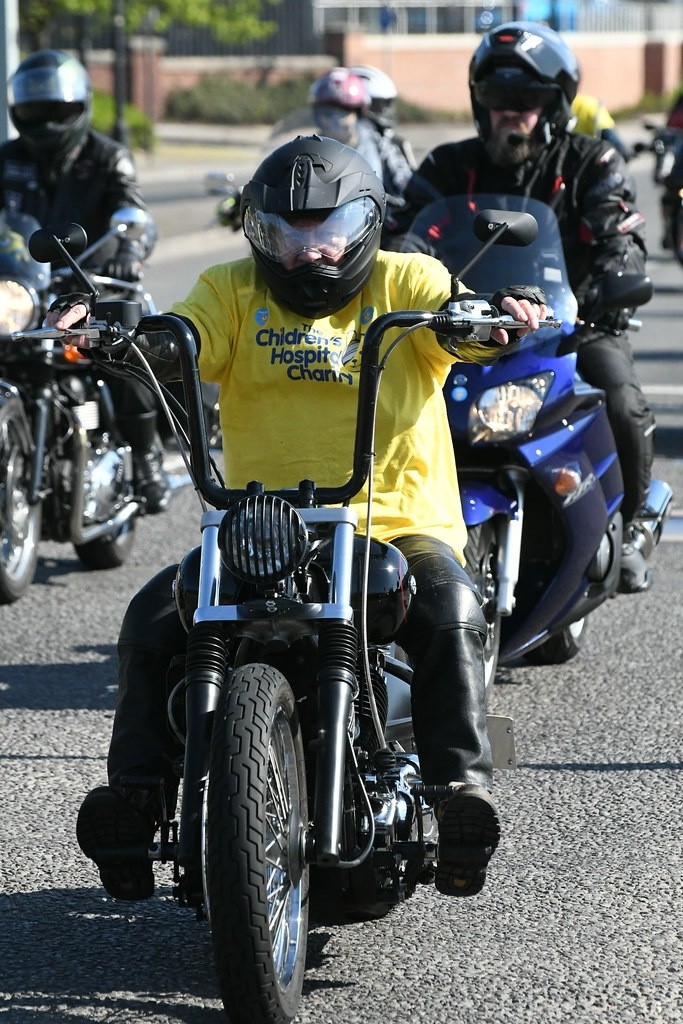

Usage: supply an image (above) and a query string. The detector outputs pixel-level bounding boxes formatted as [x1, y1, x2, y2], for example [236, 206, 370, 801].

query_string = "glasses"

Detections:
[475, 77, 560, 111]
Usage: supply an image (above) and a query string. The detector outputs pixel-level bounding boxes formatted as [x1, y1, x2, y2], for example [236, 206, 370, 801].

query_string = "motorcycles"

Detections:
[13, 206, 565, 1024]
[373, 190, 672, 677]
[0, 198, 155, 600]
[643, 118, 683, 265]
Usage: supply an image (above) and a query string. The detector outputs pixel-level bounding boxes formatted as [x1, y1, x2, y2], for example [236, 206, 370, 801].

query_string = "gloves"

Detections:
[579, 277, 635, 336]
[104, 250, 143, 292]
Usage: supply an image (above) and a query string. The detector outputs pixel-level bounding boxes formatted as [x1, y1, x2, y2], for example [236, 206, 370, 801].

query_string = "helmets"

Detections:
[9, 49, 92, 156]
[468, 22, 579, 151]
[309, 68, 369, 124]
[241, 135, 384, 319]
[352, 68, 396, 108]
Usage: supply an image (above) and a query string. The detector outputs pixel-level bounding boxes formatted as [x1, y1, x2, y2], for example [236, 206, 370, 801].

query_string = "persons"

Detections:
[0, 47, 173, 517]
[213, 61, 417, 255]
[653, 86, 683, 248]
[568, 84, 630, 165]
[50, 131, 555, 904]
[377, 19, 657, 596]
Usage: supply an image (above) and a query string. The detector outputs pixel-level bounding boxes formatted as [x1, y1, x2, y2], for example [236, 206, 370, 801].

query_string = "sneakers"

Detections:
[434, 785, 500, 897]
[78, 787, 154, 901]
[136, 445, 171, 514]
[620, 542, 653, 592]
